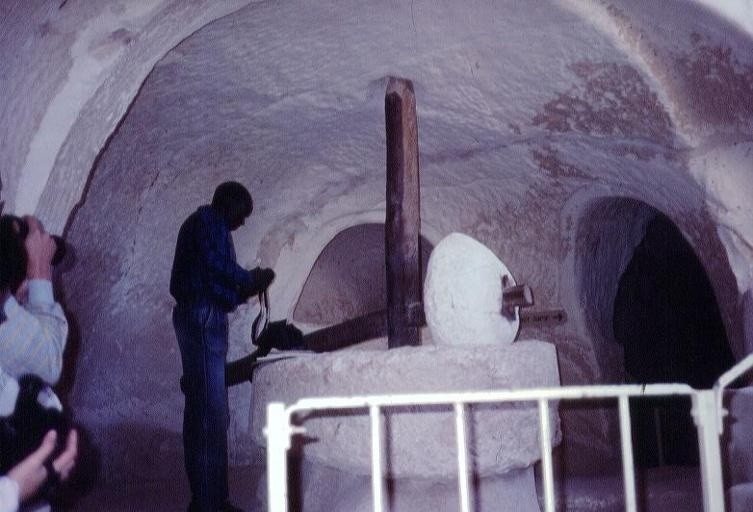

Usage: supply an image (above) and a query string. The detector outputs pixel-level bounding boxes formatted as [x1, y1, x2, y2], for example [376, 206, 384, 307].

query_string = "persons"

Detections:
[168, 180, 275, 512]
[0, 426, 80, 512]
[0, 180, 72, 418]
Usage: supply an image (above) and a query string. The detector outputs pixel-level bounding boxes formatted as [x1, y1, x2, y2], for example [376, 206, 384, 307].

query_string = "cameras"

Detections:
[0, 214, 66, 265]
